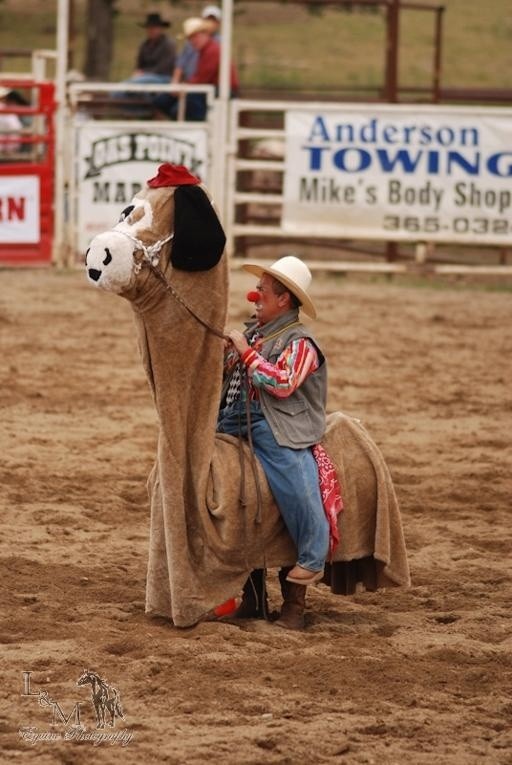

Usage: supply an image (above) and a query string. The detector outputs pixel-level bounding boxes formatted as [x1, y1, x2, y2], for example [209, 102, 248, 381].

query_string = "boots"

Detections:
[236, 568, 307, 629]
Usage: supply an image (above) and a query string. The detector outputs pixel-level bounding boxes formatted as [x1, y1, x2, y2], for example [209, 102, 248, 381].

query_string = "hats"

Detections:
[243, 256, 317, 320]
[137, 6, 220, 40]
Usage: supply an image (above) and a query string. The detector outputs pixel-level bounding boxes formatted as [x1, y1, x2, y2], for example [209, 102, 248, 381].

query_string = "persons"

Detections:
[109, 4, 241, 120]
[0, 88, 34, 154]
